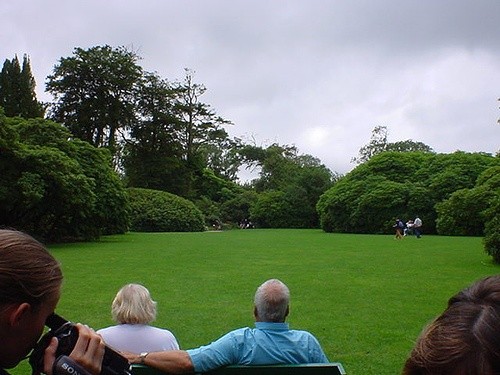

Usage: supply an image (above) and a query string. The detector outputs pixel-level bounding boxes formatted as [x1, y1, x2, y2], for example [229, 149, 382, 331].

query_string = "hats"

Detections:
[395, 219, 399, 221]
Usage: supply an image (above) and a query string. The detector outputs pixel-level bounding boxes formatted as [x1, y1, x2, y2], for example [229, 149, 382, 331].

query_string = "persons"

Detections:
[402, 275, 500, 375]
[239, 218, 254, 229]
[212, 219, 220, 230]
[0, 228, 105, 375]
[94, 283, 180, 354]
[403, 219, 421, 237]
[392, 219, 404, 240]
[118, 279, 330, 374]
[413, 215, 422, 238]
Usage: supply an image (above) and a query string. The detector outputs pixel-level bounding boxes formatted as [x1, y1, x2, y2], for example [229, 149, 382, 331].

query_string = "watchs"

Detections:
[139, 351, 148, 360]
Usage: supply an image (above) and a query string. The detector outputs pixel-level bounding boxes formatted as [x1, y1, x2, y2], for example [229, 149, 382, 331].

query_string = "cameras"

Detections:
[26, 312, 130, 375]
